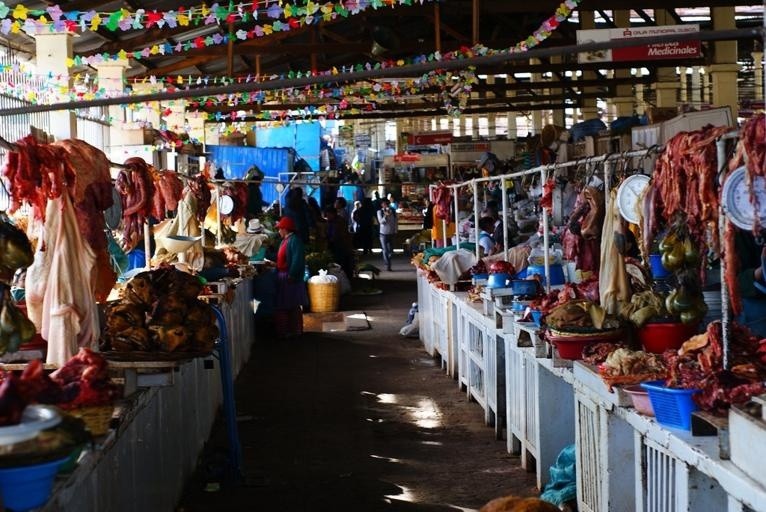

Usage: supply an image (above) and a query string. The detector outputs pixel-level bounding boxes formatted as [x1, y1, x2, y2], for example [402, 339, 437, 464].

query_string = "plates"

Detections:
[218, 195, 235, 215]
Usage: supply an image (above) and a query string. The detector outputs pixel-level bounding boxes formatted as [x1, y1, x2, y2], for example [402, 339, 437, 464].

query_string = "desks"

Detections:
[0, 260, 260, 510]
[408, 255, 766, 511]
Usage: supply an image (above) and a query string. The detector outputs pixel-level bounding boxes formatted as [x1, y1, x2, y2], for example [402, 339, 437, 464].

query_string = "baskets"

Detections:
[639, 377, 705, 431]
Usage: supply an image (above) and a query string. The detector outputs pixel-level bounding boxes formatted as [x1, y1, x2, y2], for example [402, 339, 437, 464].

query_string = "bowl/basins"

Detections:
[487, 273, 512, 288]
[641, 323, 698, 355]
[0, 408, 88, 508]
[624, 384, 653, 415]
[547, 335, 614, 359]
[512, 279, 538, 294]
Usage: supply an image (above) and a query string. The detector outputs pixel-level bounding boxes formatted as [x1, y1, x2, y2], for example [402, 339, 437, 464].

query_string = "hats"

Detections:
[275, 216, 295, 230]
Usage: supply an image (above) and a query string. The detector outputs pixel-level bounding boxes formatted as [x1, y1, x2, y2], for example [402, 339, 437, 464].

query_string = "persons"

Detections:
[242, 164, 397, 315]
[478, 199, 503, 256]
[10, 266, 27, 301]
[735, 226, 766, 338]
